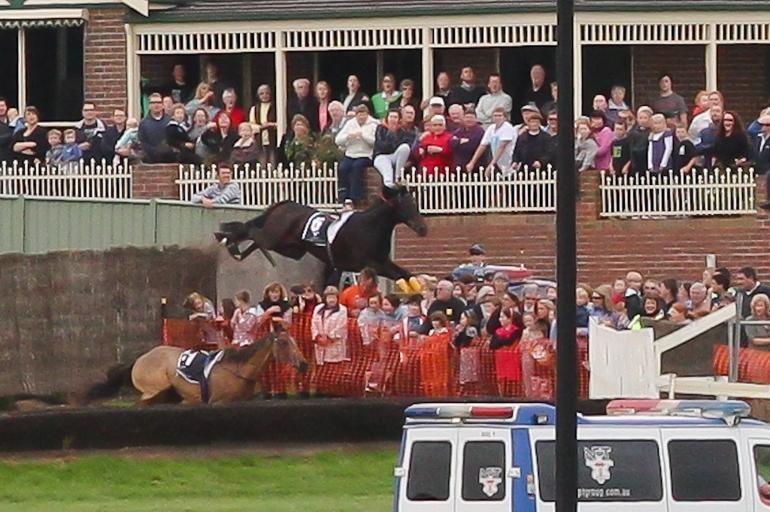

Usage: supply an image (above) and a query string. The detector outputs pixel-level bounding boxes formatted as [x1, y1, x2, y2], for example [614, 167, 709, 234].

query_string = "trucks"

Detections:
[393, 397, 769, 511]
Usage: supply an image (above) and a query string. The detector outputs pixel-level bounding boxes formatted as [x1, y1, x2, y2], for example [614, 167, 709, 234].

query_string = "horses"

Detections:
[86, 328, 309, 405]
[213, 181, 428, 295]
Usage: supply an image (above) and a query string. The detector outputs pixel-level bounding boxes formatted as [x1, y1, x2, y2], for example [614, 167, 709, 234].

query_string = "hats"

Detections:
[592, 285, 615, 312]
[469, 243, 487, 256]
[320, 285, 339, 295]
[520, 105, 540, 114]
[429, 97, 445, 107]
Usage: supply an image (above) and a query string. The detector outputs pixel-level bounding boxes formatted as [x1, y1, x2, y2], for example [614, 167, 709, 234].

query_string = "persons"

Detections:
[451, 242, 485, 280]
[186, 264, 770, 400]
[575, 74, 769, 213]
[0, 63, 557, 210]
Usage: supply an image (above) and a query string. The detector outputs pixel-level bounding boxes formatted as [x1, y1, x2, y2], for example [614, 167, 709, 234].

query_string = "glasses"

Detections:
[645, 286, 656, 290]
[592, 295, 602, 300]
[523, 296, 536, 301]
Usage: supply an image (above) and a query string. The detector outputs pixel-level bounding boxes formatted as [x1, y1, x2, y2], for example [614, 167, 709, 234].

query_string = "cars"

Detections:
[454, 259, 560, 296]
